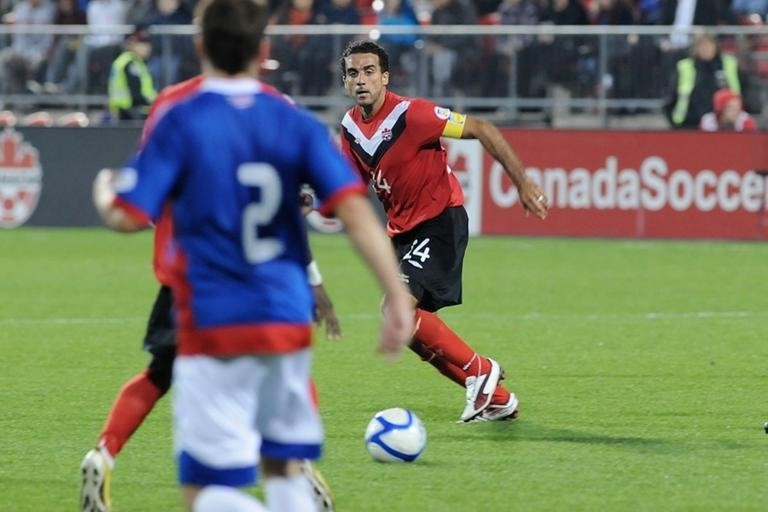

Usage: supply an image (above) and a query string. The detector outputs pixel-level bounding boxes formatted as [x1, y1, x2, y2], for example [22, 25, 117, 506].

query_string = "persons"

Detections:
[423, 2, 767, 124]
[93, 0, 414, 512]
[662, 31, 747, 130]
[81, 0, 342, 512]
[295, 41, 550, 426]
[107, 31, 160, 125]
[2, 3, 203, 112]
[255, 2, 424, 112]
[697, 88, 758, 130]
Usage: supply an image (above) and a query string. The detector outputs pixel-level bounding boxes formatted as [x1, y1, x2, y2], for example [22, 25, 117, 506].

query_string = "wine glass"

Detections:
[537, 195, 543, 202]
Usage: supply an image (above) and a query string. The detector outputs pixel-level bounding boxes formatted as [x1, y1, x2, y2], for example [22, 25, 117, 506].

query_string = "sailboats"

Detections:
[365, 408, 427, 464]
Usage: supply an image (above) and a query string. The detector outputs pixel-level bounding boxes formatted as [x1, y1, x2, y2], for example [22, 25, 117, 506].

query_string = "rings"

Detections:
[537, 195, 543, 202]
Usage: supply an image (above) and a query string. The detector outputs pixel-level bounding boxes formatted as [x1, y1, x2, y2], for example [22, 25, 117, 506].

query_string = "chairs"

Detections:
[80, 449, 112, 512]
[468, 392, 519, 421]
[457, 357, 505, 422]
